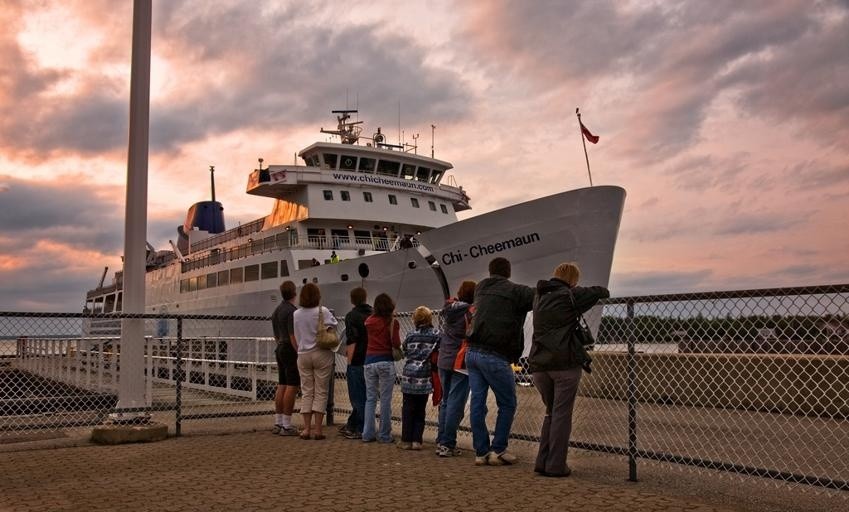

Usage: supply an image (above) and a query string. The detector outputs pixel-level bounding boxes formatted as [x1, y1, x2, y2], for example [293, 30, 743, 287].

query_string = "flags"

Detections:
[578, 117, 600, 144]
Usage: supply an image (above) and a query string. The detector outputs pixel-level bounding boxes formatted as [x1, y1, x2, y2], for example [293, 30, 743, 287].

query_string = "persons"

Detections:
[333, 233, 340, 249]
[272, 280, 299, 436]
[402, 306, 441, 450]
[318, 229, 325, 249]
[336, 287, 373, 439]
[400, 234, 413, 248]
[465, 256, 534, 465]
[530, 263, 611, 478]
[294, 282, 338, 440]
[437, 280, 477, 457]
[364, 293, 401, 443]
[311, 258, 320, 266]
[331, 251, 339, 263]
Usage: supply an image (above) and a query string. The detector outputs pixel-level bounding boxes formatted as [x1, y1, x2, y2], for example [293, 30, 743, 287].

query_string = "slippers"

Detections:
[299, 431, 310, 439]
[314, 432, 326, 440]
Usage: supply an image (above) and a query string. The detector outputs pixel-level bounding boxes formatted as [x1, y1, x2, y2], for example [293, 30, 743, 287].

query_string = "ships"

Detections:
[83, 110, 626, 385]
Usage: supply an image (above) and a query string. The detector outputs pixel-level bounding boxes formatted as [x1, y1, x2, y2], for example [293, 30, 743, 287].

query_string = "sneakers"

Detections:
[488, 451, 518, 465]
[344, 430, 362, 439]
[336, 423, 349, 432]
[548, 466, 571, 477]
[439, 445, 463, 457]
[474, 454, 489, 465]
[279, 426, 302, 436]
[272, 424, 281, 434]
[411, 441, 423, 450]
[396, 440, 411, 450]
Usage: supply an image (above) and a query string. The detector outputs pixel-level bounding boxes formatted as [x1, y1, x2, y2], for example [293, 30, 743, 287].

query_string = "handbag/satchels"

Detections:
[315, 305, 340, 349]
[389, 317, 404, 361]
[567, 288, 595, 346]
